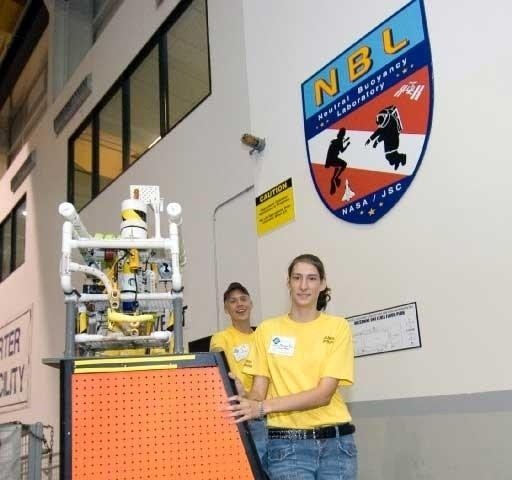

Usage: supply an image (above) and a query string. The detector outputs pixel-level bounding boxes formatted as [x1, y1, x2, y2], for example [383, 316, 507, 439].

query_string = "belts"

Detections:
[268, 423, 355, 439]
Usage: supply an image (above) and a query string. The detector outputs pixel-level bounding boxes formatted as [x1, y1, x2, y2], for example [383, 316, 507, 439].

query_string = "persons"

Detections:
[211, 282, 269, 476]
[220, 251, 360, 480]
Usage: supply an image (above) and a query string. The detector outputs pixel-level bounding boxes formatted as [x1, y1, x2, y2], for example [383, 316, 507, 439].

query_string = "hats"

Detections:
[224, 282, 249, 302]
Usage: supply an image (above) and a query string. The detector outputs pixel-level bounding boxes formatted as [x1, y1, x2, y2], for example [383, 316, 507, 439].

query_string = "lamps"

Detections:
[240, 134, 266, 151]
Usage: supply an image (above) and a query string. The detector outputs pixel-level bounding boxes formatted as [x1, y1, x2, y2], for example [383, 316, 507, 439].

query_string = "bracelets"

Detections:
[258, 400, 264, 423]
